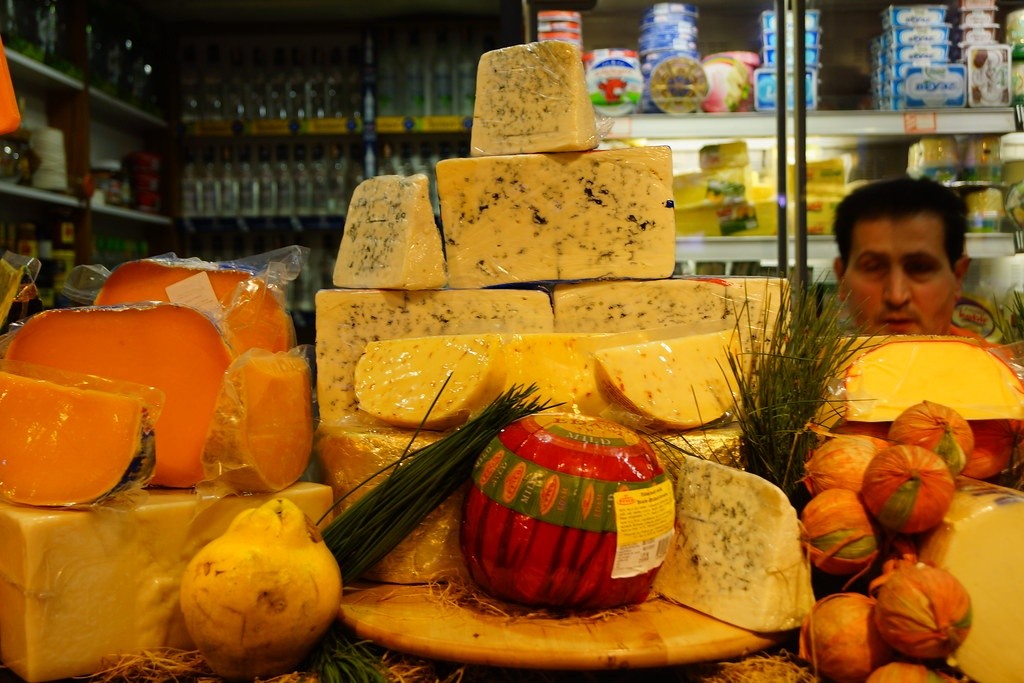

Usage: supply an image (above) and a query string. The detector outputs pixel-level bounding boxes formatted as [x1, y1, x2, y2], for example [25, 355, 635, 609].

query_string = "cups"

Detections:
[30, 128, 67, 191]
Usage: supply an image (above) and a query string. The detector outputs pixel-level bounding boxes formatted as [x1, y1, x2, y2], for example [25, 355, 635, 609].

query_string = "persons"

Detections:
[815, 175, 1006, 349]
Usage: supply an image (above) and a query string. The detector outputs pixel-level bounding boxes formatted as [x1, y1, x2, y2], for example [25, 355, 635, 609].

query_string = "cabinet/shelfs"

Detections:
[585, 104, 1018, 262]
[0, 0, 177, 309]
[170, 0, 538, 324]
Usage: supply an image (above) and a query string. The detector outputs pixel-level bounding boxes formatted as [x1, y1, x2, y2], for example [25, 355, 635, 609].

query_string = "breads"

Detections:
[0, 40, 1024, 683]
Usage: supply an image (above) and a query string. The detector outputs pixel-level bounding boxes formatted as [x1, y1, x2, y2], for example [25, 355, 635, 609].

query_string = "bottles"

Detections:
[179, 25, 498, 299]
